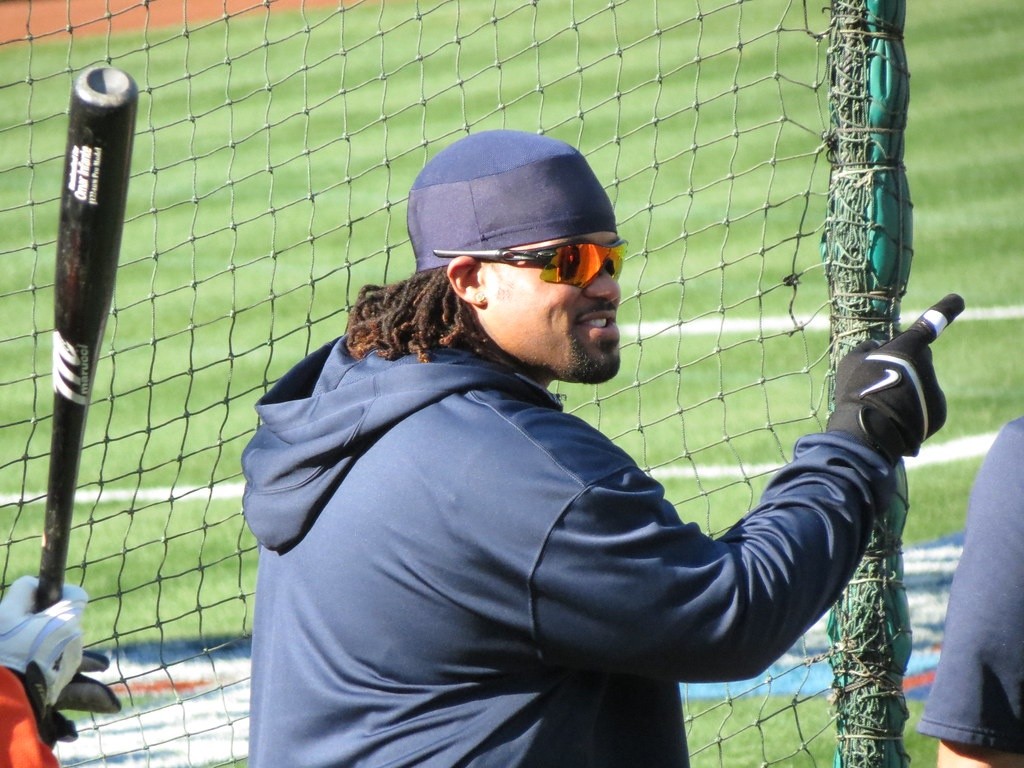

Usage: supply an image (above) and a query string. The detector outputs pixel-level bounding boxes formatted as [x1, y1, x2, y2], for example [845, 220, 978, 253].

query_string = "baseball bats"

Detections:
[30, 64, 140, 617]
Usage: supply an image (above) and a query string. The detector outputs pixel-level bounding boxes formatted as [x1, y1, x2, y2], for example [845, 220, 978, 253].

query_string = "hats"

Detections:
[406, 130, 618, 273]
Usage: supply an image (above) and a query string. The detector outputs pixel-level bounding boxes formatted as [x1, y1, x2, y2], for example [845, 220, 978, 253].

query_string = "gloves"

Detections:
[0, 575, 121, 751]
[825, 294, 966, 467]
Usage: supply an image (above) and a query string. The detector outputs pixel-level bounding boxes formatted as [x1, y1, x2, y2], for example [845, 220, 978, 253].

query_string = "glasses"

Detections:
[434, 240, 629, 289]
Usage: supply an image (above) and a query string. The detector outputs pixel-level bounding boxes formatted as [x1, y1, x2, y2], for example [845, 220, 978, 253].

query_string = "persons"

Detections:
[240, 130, 966, 768]
[916, 414, 1024, 768]
[0, 574, 121, 768]
